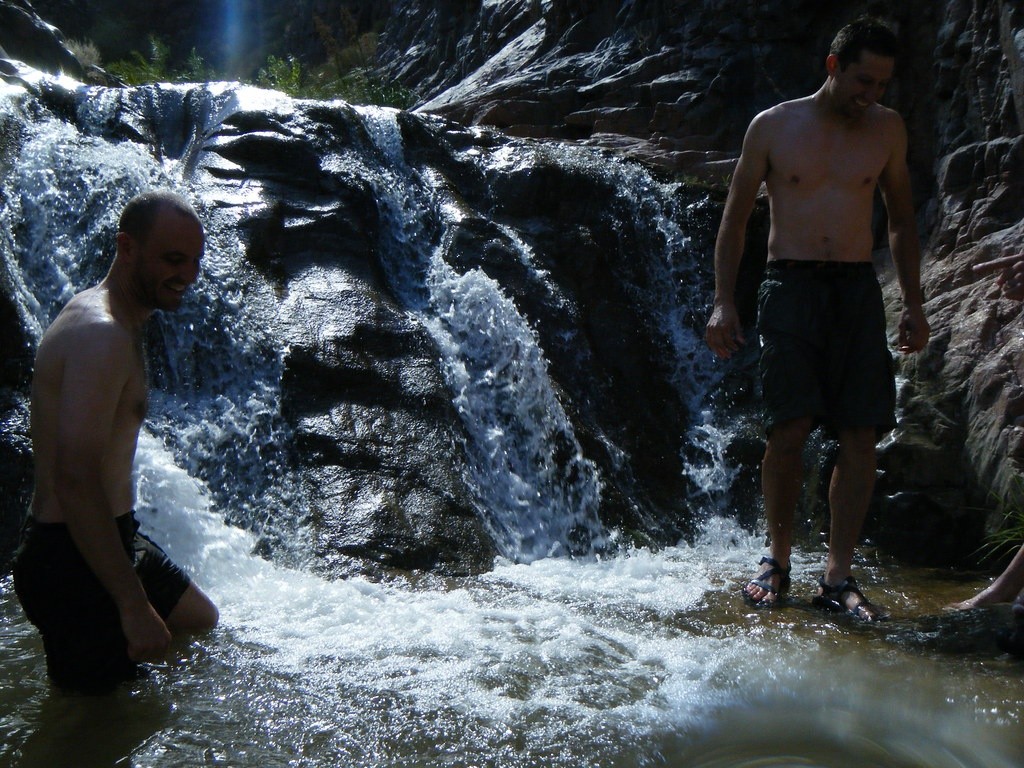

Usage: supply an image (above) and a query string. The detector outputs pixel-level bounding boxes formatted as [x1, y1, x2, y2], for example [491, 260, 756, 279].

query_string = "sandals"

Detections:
[743, 555, 792, 608]
[811, 572, 888, 622]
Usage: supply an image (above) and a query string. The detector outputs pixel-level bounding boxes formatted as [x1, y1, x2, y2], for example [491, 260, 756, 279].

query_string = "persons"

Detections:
[13, 193, 220, 679]
[705, 22, 930, 617]
[969, 544, 1024, 614]
